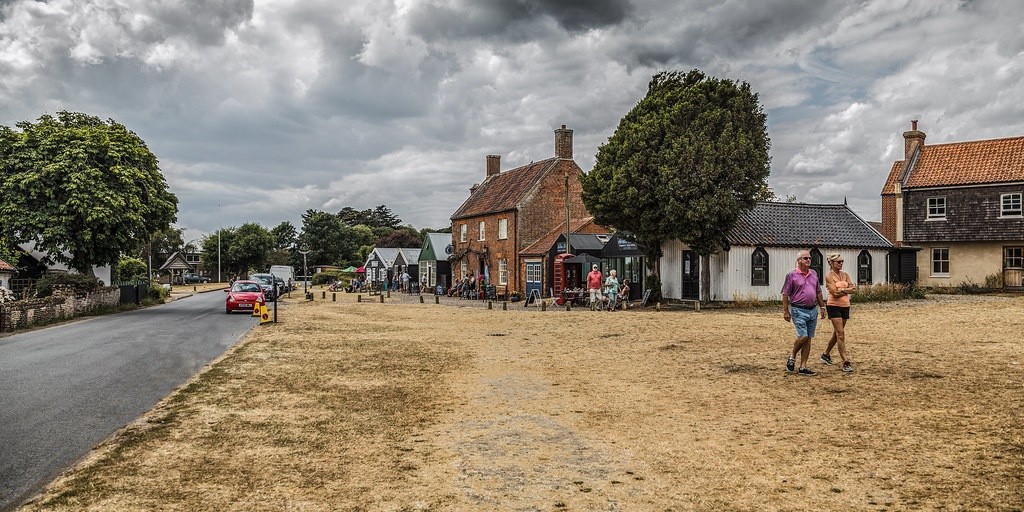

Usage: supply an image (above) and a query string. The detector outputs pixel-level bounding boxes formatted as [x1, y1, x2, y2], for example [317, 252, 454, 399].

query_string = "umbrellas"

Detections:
[560, 253, 606, 282]
[343, 265, 365, 273]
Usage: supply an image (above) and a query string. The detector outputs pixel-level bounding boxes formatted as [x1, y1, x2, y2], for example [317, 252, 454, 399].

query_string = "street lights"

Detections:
[562, 173, 572, 254]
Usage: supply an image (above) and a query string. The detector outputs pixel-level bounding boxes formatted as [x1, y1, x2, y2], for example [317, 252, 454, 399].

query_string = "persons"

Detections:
[384, 271, 412, 293]
[821, 252, 856, 372]
[288, 277, 292, 291]
[447, 274, 494, 299]
[421, 273, 427, 293]
[229, 276, 234, 286]
[781, 249, 827, 376]
[587, 264, 630, 311]
[327, 274, 372, 293]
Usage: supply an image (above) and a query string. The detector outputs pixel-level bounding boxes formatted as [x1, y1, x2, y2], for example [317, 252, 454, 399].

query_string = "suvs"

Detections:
[184, 273, 211, 283]
[251, 274, 275, 302]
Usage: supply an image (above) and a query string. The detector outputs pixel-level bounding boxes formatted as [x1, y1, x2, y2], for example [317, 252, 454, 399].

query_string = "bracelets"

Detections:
[820, 307, 826, 310]
[841, 288, 844, 292]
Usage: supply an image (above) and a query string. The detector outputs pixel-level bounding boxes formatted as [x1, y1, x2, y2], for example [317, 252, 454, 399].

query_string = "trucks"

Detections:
[270, 265, 295, 290]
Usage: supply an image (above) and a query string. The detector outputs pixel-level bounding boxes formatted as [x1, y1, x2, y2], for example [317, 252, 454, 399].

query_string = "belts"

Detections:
[791, 304, 816, 310]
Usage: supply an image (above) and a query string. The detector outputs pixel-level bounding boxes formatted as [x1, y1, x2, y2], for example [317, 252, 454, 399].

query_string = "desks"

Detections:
[565, 290, 586, 307]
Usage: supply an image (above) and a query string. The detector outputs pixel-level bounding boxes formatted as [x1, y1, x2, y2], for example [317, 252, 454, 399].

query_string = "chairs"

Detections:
[586, 289, 631, 309]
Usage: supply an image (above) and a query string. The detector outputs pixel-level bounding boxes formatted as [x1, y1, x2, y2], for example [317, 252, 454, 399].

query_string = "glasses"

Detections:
[594, 267, 597, 268]
[803, 257, 812, 260]
[832, 260, 844, 264]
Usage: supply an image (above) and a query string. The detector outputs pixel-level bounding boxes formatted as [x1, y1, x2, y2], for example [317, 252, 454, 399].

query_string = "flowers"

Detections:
[510, 290, 522, 296]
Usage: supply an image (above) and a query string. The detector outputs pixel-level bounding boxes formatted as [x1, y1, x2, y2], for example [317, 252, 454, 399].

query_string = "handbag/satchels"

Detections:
[605, 278, 611, 293]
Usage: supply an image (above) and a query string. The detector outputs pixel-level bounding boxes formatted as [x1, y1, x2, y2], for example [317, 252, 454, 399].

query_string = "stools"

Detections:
[547, 298, 560, 307]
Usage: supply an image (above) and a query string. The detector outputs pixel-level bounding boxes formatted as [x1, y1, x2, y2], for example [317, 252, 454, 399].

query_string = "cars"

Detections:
[274, 276, 285, 296]
[224, 280, 265, 314]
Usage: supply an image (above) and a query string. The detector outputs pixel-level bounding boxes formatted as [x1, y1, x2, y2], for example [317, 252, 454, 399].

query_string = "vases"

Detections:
[425, 288, 433, 292]
[511, 295, 519, 302]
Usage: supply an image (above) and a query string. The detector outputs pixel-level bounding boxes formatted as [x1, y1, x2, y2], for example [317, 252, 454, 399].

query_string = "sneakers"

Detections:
[786, 356, 796, 372]
[820, 353, 833, 365]
[799, 368, 817, 376]
[842, 361, 853, 372]
